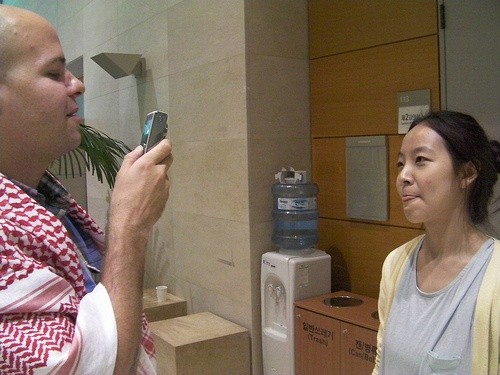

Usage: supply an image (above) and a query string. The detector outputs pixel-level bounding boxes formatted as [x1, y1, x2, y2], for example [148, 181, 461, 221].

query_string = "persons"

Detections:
[0, 3, 173, 375]
[371, 110, 500, 375]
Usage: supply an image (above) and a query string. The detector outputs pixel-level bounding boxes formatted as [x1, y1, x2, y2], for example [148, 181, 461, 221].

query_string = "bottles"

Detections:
[271, 182, 319, 250]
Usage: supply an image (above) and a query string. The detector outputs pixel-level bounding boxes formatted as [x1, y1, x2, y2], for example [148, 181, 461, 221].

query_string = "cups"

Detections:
[155, 285, 168, 302]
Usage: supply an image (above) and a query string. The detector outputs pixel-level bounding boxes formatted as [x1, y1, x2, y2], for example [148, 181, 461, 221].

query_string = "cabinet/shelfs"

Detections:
[294, 307, 379, 375]
[143, 287, 250, 375]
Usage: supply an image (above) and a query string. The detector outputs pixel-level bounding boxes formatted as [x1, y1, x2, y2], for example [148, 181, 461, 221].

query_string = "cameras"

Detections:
[139, 110, 169, 157]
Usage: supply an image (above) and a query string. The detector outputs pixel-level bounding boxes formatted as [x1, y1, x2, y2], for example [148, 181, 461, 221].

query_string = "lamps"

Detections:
[91, 52, 146, 80]
